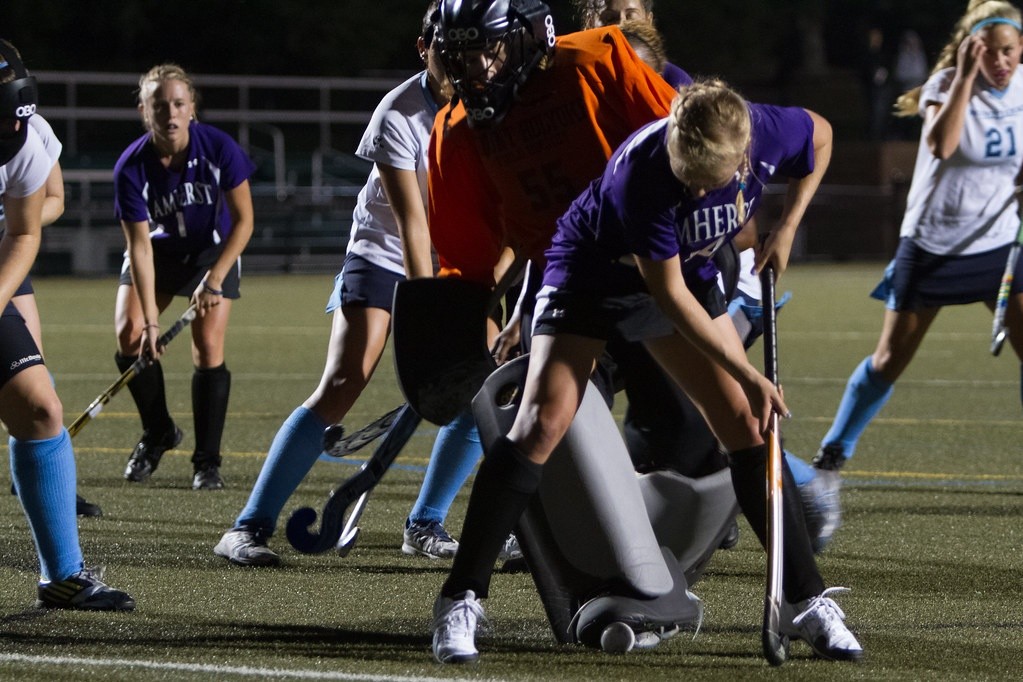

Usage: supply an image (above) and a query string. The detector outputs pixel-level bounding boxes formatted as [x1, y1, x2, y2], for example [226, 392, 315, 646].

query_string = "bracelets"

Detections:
[200, 279, 224, 295]
[143, 324, 159, 330]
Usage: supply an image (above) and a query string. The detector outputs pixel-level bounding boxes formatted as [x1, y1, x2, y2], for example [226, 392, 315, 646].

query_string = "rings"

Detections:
[206, 301, 211, 307]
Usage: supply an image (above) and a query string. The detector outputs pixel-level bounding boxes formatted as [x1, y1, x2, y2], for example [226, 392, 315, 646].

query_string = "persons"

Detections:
[809, 1, 1022, 475]
[211, 0, 848, 566]
[857, 27, 930, 91]
[1, 39, 139, 613]
[111, 64, 257, 488]
[431, 74, 868, 662]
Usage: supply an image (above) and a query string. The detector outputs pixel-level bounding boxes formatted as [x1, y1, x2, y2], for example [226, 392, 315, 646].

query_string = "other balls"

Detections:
[600, 622, 635, 655]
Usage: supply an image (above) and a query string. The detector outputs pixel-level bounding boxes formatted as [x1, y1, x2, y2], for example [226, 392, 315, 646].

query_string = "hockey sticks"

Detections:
[10, 301, 199, 497]
[989, 216, 1023, 357]
[320, 400, 407, 457]
[334, 250, 525, 558]
[755, 230, 791, 665]
[284, 402, 422, 557]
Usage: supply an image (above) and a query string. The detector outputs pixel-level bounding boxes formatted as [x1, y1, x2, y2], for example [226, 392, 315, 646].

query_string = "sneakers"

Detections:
[718, 523, 738, 548]
[36, 562, 135, 614]
[192, 453, 224, 492]
[799, 470, 840, 546]
[212, 511, 279, 566]
[74, 496, 103, 515]
[431, 582, 489, 661]
[401, 515, 460, 556]
[778, 587, 863, 663]
[502, 532, 526, 559]
[122, 421, 184, 482]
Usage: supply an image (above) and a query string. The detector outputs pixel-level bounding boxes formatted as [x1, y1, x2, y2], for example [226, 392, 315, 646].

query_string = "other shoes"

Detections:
[815, 441, 847, 477]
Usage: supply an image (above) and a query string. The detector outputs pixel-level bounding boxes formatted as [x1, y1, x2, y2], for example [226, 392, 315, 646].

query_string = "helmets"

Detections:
[430, 0, 555, 134]
